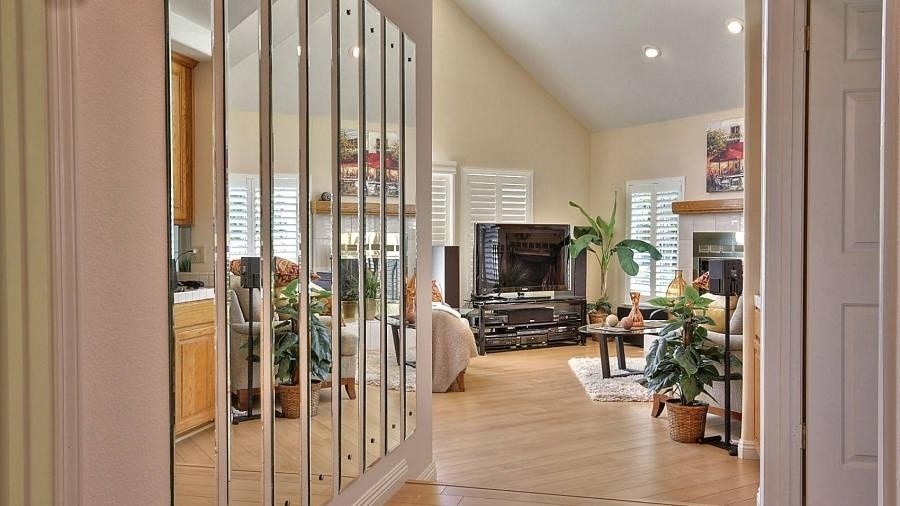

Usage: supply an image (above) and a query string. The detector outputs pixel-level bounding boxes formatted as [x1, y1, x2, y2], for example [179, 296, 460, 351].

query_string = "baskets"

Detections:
[588, 311, 617, 342]
[278, 379, 322, 420]
[665, 398, 709, 443]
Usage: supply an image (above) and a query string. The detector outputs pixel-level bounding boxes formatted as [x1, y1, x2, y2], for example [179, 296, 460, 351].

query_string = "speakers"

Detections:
[709, 259, 743, 295]
[571, 249, 587, 299]
[432, 246, 459, 309]
[494, 306, 553, 325]
[240, 257, 261, 288]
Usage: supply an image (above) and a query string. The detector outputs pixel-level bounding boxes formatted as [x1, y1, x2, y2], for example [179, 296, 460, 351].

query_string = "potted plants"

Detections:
[649, 286, 716, 443]
[571, 204, 644, 329]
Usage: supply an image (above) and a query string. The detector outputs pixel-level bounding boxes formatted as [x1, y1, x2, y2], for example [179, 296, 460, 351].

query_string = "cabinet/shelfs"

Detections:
[460, 291, 588, 355]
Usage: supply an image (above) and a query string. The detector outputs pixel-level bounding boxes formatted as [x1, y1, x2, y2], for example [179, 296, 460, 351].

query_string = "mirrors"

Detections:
[163, 0, 417, 506]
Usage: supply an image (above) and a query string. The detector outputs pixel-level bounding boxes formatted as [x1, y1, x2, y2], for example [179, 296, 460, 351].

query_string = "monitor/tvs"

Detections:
[474, 223, 571, 302]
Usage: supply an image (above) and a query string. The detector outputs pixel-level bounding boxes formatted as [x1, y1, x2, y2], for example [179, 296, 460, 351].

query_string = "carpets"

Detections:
[569, 353, 680, 405]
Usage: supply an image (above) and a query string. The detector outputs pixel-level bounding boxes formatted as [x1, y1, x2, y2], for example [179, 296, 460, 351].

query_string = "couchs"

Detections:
[682, 294, 742, 420]
